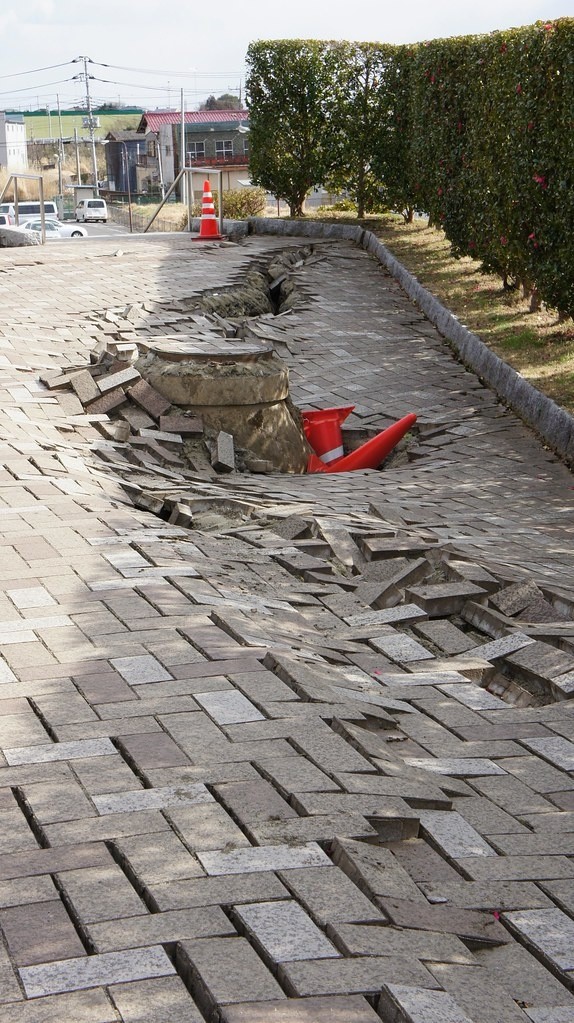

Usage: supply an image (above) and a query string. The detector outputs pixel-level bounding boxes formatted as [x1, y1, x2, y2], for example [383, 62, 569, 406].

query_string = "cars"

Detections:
[0, 215, 88, 237]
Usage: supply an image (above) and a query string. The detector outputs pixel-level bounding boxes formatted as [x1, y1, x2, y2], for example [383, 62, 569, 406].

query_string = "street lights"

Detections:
[54, 154, 62, 195]
[100, 140, 133, 232]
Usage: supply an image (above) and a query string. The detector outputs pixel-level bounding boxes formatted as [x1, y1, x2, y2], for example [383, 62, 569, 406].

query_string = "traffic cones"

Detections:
[303, 405, 355, 469]
[307, 414, 417, 473]
[198, 180, 221, 237]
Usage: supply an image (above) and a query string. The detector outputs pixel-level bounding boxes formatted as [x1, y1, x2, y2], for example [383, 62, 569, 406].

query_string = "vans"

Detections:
[75, 199, 107, 223]
[0, 201, 59, 221]
